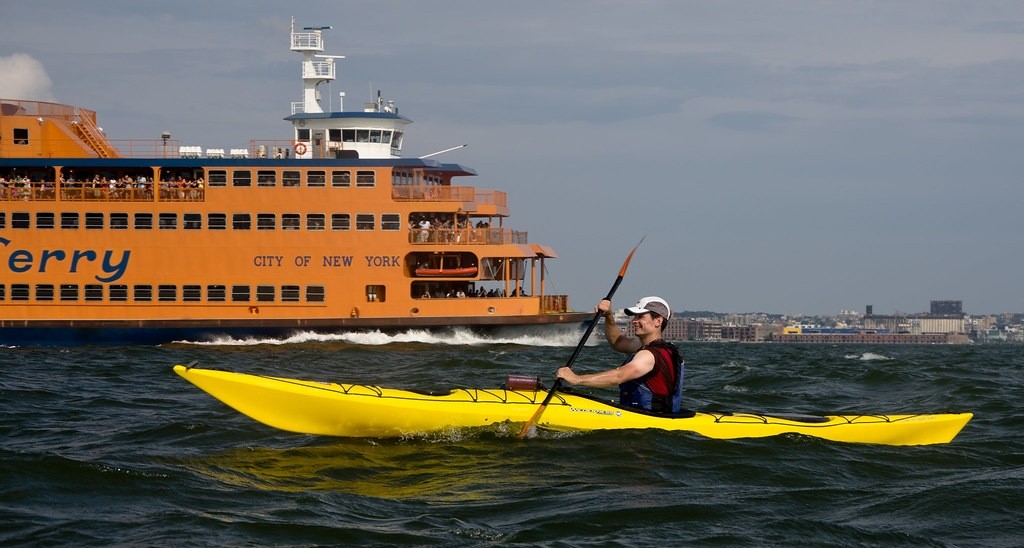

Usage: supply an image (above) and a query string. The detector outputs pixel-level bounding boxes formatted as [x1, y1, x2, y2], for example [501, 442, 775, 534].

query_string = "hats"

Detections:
[624, 296, 670, 322]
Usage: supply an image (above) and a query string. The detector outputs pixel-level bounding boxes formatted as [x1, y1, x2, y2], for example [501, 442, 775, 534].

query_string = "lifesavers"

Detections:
[294, 142, 307, 155]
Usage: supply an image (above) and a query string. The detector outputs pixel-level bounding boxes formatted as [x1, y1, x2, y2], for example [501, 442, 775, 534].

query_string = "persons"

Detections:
[59, 172, 153, 199]
[408, 215, 489, 243]
[159, 176, 204, 202]
[0, 172, 55, 201]
[556, 296, 683, 414]
[423, 286, 528, 298]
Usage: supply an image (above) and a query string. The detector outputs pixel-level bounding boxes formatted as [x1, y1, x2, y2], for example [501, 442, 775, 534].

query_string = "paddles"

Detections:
[515, 233, 649, 441]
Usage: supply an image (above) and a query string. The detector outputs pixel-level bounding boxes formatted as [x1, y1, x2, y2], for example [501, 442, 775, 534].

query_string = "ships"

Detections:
[0, 15, 599, 346]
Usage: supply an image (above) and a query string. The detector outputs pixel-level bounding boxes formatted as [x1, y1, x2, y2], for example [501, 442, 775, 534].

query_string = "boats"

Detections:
[173, 362, 973, 444]
